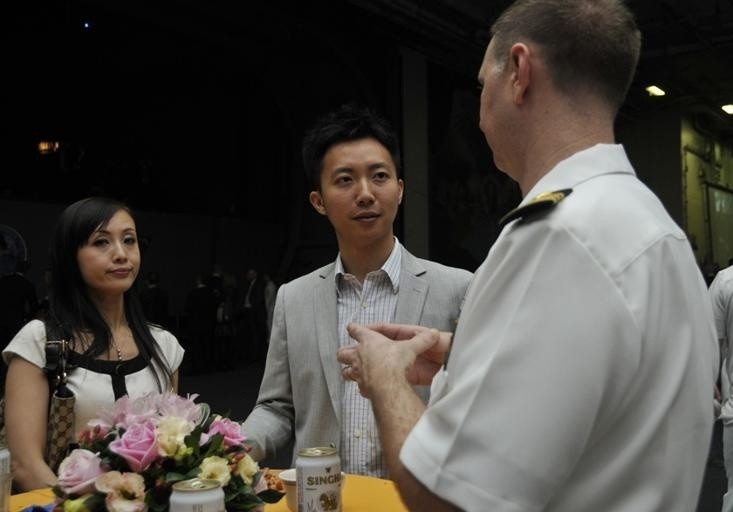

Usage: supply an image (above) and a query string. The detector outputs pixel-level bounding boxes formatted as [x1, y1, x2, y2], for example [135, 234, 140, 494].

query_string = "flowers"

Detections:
[47, 390, 287, 512]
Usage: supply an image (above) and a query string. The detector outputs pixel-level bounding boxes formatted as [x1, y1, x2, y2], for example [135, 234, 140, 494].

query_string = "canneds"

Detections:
[295, 447, 342, 512]
[168, 477, 228, 512]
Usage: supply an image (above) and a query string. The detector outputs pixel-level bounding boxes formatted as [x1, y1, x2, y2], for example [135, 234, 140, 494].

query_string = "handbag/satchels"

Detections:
[0, 386, 75, 496]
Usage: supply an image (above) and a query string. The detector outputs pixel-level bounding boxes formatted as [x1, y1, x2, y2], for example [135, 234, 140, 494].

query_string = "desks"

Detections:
[0, 469, 412, 512]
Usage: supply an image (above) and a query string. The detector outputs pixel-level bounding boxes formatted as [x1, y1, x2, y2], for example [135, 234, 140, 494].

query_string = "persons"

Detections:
[0, 249, 272, 403]
[236, 100, 472, 482]
[332, 0, 717, 512]
[0, 195, 188, 491]
[709, 264, 733, 510]
